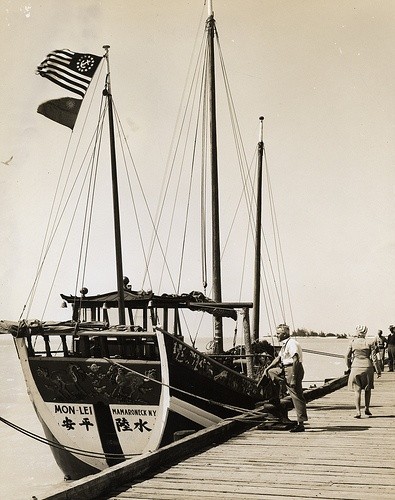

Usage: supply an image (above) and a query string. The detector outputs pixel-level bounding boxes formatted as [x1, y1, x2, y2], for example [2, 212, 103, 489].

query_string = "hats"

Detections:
[356, 325, 368, 336]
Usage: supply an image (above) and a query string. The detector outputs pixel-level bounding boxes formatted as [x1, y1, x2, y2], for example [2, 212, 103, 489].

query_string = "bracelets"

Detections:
[292, 374, 297, 377]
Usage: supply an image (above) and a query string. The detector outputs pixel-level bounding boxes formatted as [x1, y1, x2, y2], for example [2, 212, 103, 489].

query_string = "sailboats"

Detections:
[0, 0, 298, 476]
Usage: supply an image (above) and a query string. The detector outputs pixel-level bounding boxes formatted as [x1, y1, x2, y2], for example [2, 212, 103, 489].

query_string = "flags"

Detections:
[37, 97, 83, 130]
[35, 48, 103, 98]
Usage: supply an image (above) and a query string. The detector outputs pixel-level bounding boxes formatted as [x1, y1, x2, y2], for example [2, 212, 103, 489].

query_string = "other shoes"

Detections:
[291, 424, 304, 432]
[354, 414, 361, 418]
[388, 369, 393, 372]
[268, 398, 281, 405]
[365, 410, 372, 415]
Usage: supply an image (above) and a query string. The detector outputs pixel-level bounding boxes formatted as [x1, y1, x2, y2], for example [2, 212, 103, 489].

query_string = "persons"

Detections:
[387, 324, 395, 372]
[263, 324, 308, 432]
[370, 329, 388, 371]
[346, 325, 382, 418]
